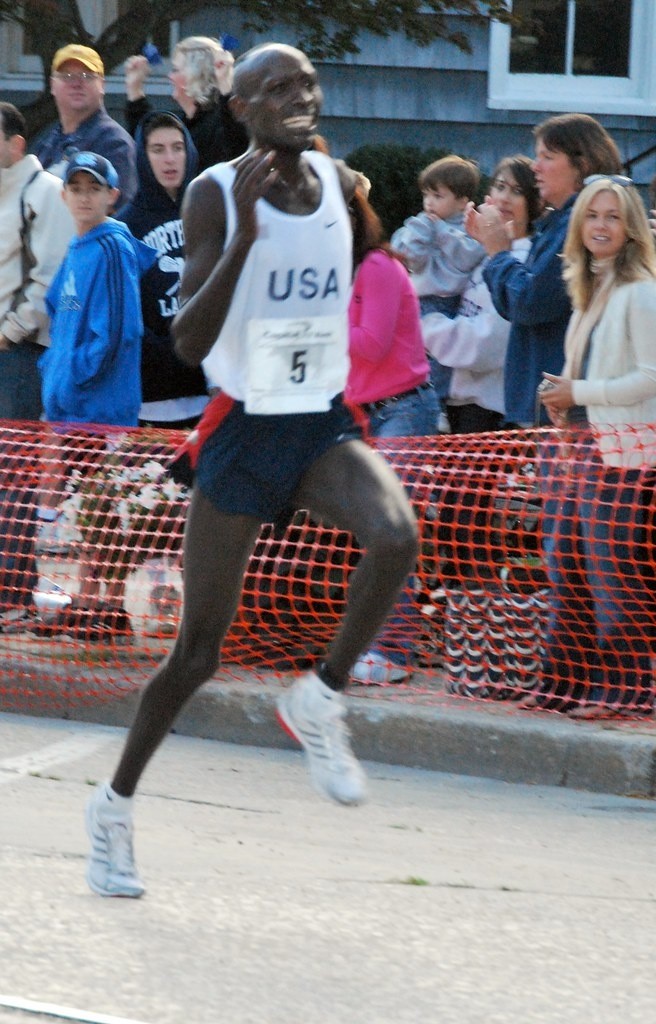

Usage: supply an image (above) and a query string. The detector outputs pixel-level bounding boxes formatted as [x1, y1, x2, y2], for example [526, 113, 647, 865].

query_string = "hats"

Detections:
[52, 43, 104, 78]
[61, 151, 119, 189]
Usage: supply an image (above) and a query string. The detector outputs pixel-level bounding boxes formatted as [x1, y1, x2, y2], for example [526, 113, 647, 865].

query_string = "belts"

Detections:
[359, 380, 433, 414]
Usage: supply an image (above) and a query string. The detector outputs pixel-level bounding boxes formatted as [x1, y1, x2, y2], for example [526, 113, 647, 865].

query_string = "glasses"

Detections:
[583, 173, 634, 187]
[51, 70, 98, 84]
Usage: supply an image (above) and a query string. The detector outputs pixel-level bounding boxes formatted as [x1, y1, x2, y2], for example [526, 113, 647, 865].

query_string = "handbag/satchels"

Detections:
[7, 280, 39, 353]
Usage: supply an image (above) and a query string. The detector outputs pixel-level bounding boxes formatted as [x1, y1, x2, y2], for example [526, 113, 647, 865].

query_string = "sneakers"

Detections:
[26, 602, 132, 648]
[273, 675, 368, 807]
[349, 654, 411, 684]
[82, 784, 147, 897]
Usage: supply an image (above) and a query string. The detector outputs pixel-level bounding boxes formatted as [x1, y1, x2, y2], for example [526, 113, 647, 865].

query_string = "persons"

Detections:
[390, 154, 486, 434]
[462, 116, 656, 721]
[418, 156, 545, 651]
[343, 190, 450, 684]
[0, 35, 254, 641]
[84, 44, 419, 895]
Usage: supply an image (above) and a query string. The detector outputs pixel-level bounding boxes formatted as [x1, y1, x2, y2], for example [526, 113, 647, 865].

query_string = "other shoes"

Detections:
[569, 704, 645, 718]
[1, 605, 37, 634]
[521, 696, 541, 712]
